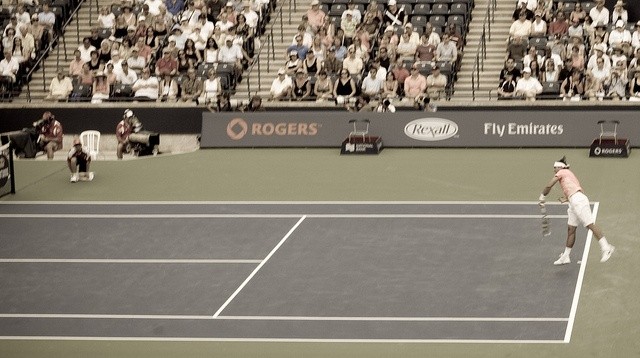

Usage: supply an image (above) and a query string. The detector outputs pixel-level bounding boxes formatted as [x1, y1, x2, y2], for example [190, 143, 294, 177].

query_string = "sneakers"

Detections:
[553, 253, 570, 265]
[89, 172, 94, 181]
[600, 245, 615, 263]
[388, 105, 396, 113]
[71, 173, 79, 181]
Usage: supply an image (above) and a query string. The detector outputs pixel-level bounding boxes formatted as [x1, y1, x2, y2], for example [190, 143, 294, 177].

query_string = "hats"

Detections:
[290, 50, 298, 56]
[612, 45, 624, 50]
[593, 46, 604, 52]
[535, 10, 542, 16]
[226, 2, 232, 6]
[241, 1, 250, 8]
[595, 20, 607, 27]
[570, 31, 583, 38]
[276, 69, 284, 74]
[346, 10, 352, 15]
[226, 35, 234, 41]
[432, 65, 440, 70]
[405, 22, 412, 28]
[523, 66, 531, 72]
[138, 15, 146, 22]
[73, 139, 82, 145]
[123, 109, 133, 117]
[92, 71, 106, 78]
[120, 3, 132, 10]
[181, 16, 188, 22]
[170, 23, 183, 33]
[616, 20, 625, 28]
[312, 0, 319, 5]
[614, 1, 625, 7]
[388, 0, 397, 5]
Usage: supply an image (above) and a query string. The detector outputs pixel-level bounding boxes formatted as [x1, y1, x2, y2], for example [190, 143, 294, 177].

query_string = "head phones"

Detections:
[50, 114, 56, 120]
[122, 109, 135, 119]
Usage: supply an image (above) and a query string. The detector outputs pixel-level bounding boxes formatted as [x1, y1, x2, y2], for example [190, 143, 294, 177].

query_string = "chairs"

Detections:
[320, 0, 334, 4]
[528, 38, 548, 56]
[197, 65, 213, 79]
[433, 27, 442, 38]
[55, 0, 68, 22]
[318, 4, 328, 15]
[598, 119, 618, 144]
[448, 15, 464, 63]
[563, 2, 575, 13]
[581, 3, 595, 13]
[539, 82, 562, 100]
[429, 15, 445, 30]
[452, 0, 471, 23]
[435, 62, 452, 101]
[370, 0, 388, 4]
[445, 26, 461, 73]
[114, 85, 130, 97]
[77, 131, 100, 181]
[99, 28, 110, 39]
[39, 0, 53, 6]
[329, 16, 341, 28]
[50, 6, 63, 33]
[330, 4, 346, 15]
[347, 118, 371, 143]
[395, 25, 405, 34]
[417, 0, 434, 4]
[367, 4, 384, 12]
[432, 4, 449, 15]
[413, 26, 423, 36]
[71, 82, 93, 102]
[434, 0, 450, 4]
[352, 0, 369, 4]
[451, 4, 467, 36]
[110, 4, 122, 17]
[403, 61, 413, 74]
[355, 4, 364, 12]
[414, 4, 431, 15]
[416, 64, 432, 77]
[334, 0, 350, 4]
[397, 0, 415, 4]
[217, 63, 237, 91]
[397, 4, 412, 14]
[411, 16, 427, 28]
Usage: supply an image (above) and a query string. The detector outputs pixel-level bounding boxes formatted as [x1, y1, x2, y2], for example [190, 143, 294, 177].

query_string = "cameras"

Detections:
[332, 95, 358, 107]
[414, 96, 431, 105]
[228, 97, 252, 109]
[381, 96, 390, 107]
[122, 130, 160, 157]
[0, 119, 49, 158]
[195, 97, 220, 106]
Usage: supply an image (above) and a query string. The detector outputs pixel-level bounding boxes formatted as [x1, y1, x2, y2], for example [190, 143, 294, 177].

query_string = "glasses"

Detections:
[341, 73, 348, 75]
[567, 58, 572, 63]
[164, 52, 170, 54]
[380, 51, 386, 53]
[596, 61, 602, 64]
[617, 64, 624, 67]
[635, 25, 640, 28]
[32, 18, 39, 21]
[633, 71, 640, 73]
[127, 30, 134, 32]
[226, 6, 233, 8]
[507, 61, 513, 64]
[512, 39, 517, 41]
[575, 6, 580, 9]
[299, 28, 305, 30]
[349, 51, 356, 54]
[297, 40, 302, 42]
[523, 71, 530, 74]
[174, 28, 181, 31]
[209, 73, 215, 76]
[354, 39, 359, 42]
[8, 32, 14, 35]
[411, 67, 417, 71]
[318, 73, 324, 76]
[442, 37, 448, 40]
[373, 61, 379, 63]
[348, 3, 353, 5]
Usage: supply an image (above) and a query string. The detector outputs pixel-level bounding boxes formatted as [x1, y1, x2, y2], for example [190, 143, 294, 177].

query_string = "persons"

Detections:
[265, 0, 459, 106]
[0, 1, 56, 84]
[114, 109, 145, 158]
[497, 1, 640, 103]
[50, 1, 271, 103]
[538, 157, 617, 268]
[68, 139, 96, 183]
[37, 112, 64, 161]
